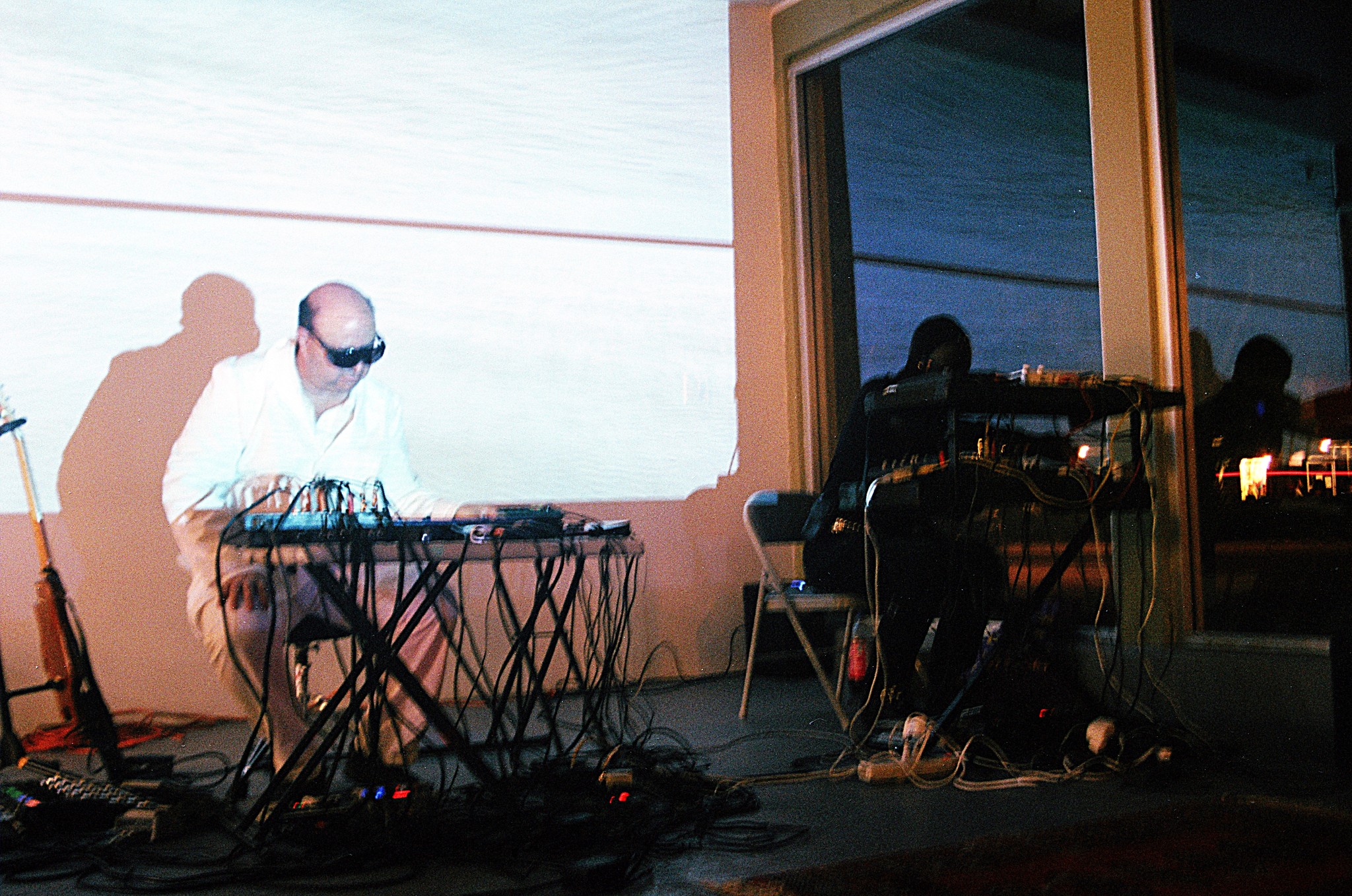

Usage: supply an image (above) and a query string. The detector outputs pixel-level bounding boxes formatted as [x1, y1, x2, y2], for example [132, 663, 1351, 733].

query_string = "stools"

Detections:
[243, 614, 354, 780]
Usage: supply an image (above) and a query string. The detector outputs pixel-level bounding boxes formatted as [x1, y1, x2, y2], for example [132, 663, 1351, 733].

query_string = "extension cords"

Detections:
[859, 749, 960, 783]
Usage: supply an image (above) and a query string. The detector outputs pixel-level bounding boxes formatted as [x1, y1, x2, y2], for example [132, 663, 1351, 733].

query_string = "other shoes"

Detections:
[283, 756, 329, 810]
[339, 743, 434, 798]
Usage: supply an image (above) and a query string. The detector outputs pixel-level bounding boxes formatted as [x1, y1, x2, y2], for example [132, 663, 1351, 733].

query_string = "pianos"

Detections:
[857, 376, 1183, 418]
[841, 456, 1178, 758]
[230, 520, 646, 888]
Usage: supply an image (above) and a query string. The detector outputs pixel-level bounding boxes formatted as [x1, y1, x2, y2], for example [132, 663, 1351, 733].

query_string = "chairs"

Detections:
[740, 486, 877, 732]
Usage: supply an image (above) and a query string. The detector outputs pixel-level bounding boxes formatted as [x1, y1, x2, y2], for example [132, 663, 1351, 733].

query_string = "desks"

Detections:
[232, 531, 652, 799]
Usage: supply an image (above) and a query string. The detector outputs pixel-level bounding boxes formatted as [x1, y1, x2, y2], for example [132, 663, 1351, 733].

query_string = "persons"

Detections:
[805, 313, 1018, 730]
[1197, 330, 1296, 543]
[160, 282, 494, 802]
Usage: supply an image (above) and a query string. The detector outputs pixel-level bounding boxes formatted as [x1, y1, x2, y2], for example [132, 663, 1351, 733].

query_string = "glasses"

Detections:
[297, 324, 385, 368]
